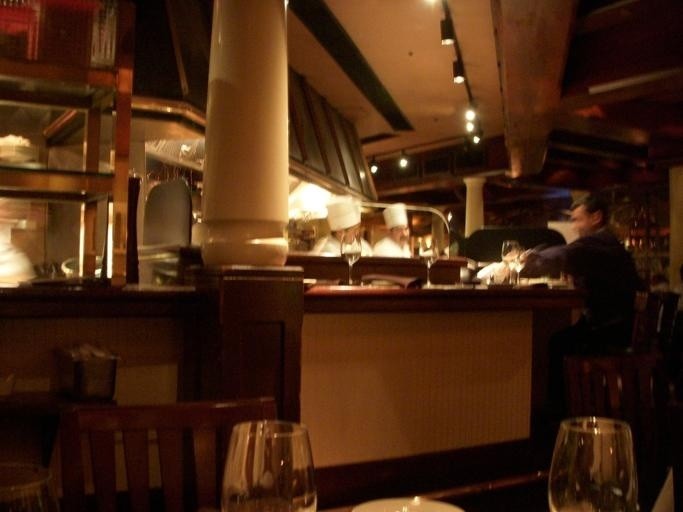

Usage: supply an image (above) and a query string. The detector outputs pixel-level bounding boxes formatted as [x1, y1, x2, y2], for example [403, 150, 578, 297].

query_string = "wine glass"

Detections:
[501, 240, 527, 286]
[419, 238, 439, 284]
[340, 231, 362, 284]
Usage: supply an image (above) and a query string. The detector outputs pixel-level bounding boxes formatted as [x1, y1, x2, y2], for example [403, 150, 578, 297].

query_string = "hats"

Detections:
[326, 195, 361, 232]
[383, 202, 408, 231]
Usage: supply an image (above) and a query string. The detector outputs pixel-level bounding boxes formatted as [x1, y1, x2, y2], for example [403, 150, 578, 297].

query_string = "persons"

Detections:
[477, 192, 637, 417]
[313, 195, 412, 259]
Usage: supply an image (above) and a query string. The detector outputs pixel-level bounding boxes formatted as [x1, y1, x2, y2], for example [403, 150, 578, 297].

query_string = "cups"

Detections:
[0, 463, 60, 511]
[221, 420, 318, 511]
[549, 417, 638, 512]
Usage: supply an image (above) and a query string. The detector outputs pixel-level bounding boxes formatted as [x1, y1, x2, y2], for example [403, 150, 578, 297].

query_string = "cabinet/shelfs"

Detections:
[0, 0, 135, 287]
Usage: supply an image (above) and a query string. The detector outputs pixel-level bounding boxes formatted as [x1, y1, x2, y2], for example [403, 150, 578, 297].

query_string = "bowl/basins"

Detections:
[0, 145, 33, 162]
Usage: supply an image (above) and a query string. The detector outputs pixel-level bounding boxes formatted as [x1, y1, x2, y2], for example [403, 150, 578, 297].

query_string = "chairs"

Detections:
[60, 397, 279, 512]
[569, 291, 680, 455]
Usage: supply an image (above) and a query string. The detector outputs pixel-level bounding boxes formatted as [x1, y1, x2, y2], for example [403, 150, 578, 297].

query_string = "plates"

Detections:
[353, 498, 464, 512]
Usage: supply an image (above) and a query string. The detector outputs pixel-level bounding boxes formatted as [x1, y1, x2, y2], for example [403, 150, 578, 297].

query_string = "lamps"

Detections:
[365, 151, 411, 176]
[440, 15, 484, 146]
